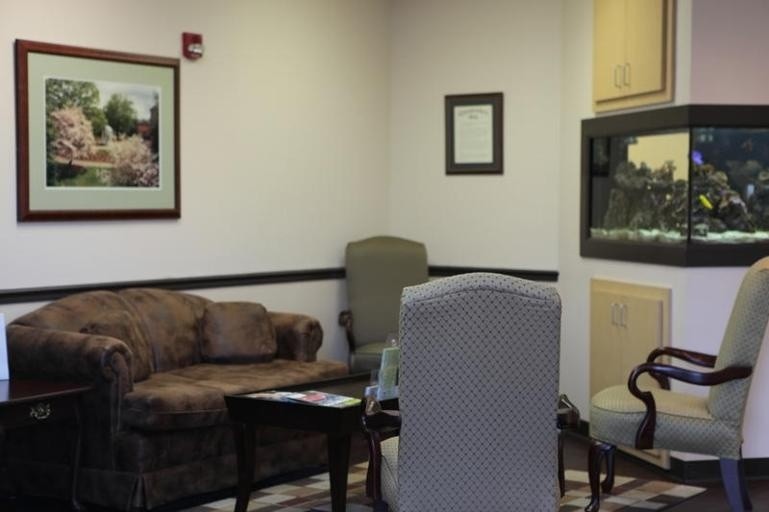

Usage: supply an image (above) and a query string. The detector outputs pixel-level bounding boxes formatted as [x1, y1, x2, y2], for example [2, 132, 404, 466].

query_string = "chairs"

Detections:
[578, 251, 768, 512]
[335, 229, 433, 375]
[360, 272, 577, 511]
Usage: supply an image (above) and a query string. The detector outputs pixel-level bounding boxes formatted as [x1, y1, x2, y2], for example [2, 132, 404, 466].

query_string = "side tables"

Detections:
[0, 375, 97, 511]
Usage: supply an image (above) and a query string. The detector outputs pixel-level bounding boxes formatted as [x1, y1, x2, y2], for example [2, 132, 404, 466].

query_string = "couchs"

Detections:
[7, 282, 349, 510]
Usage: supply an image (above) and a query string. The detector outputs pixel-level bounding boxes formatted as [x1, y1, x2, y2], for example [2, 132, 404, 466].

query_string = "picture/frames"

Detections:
[14, 37, 182, 222]
[444, 92, 503, 176]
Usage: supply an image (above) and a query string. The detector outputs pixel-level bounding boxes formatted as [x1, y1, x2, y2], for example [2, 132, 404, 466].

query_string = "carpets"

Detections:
[177, 455, 707, 512]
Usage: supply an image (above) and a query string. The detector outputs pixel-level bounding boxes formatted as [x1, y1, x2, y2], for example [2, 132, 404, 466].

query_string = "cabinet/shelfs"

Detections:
[588, 280, 671, 476]
[591, 0, 677, 109]
